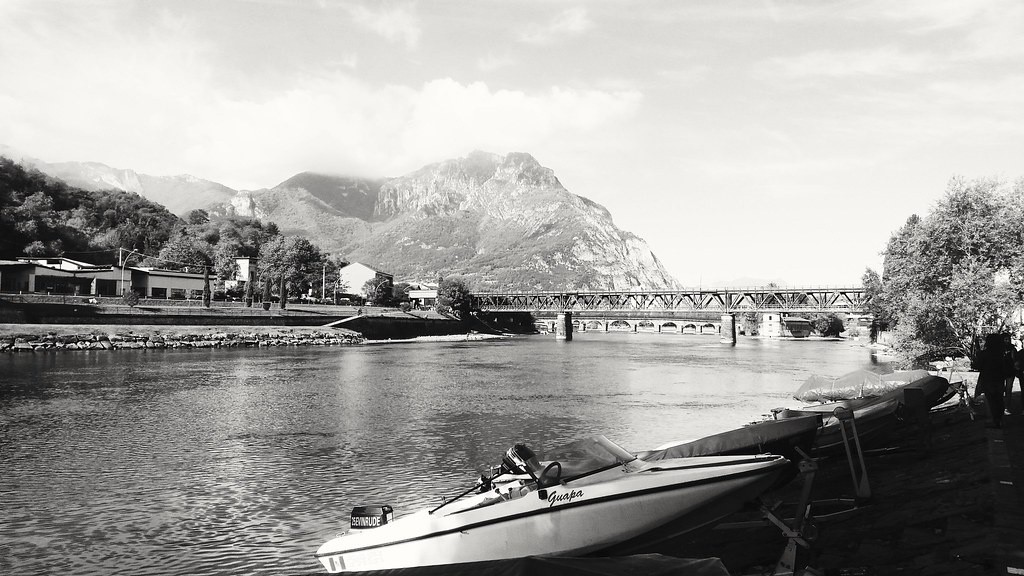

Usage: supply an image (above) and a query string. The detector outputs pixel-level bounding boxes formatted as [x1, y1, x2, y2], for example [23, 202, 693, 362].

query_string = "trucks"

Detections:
[300, 294, 320, 304]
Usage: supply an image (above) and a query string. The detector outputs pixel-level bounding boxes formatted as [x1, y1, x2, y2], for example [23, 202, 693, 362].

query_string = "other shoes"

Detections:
[1003, 408, 1012, 416]
[1019, 410, 1024, 416]
[984, 420, 1000, 428]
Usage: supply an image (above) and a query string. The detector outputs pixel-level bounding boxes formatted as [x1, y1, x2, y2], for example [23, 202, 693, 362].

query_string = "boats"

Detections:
[743, 375, 949, 459]
[311, 434, 795, 575]
[771, 381, 961, 420]
[639, 407, 824, 502]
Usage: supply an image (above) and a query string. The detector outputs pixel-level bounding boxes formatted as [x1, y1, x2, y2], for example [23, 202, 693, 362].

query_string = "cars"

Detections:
[287, 295, 298, 303]
[320, 297, 333, 305]
[365, 302, 374, 307]
[261, 295, 280, 303]
[192, 291, 203, 300]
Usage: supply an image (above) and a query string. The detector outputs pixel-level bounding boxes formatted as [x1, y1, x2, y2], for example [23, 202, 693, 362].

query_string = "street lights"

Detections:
[375, 279, 389, 299]
[122, 248, 138, 297]
[258, 263, 274, 303]
[401, 285, 412, 301]
[334, 274, 344, 305]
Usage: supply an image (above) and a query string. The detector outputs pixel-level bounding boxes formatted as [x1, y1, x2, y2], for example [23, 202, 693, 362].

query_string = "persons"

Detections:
[973, 334, 1024, 430]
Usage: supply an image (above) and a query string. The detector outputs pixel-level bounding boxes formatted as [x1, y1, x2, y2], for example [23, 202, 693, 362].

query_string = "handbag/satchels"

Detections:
[1000, 350, 1024, 378]
